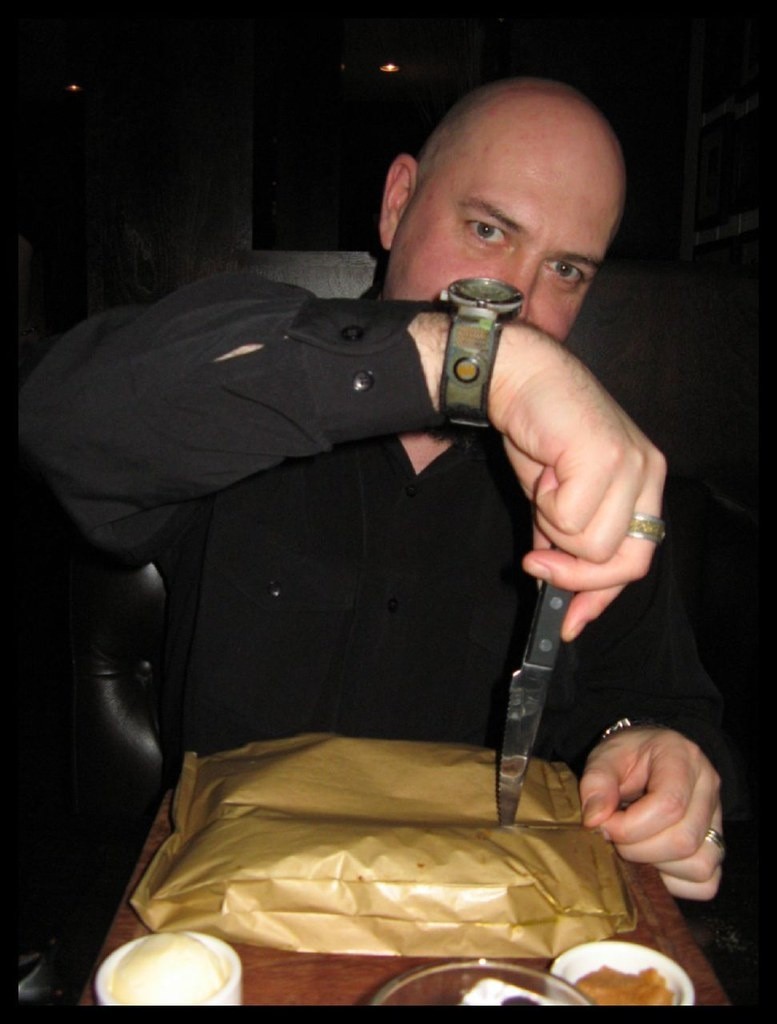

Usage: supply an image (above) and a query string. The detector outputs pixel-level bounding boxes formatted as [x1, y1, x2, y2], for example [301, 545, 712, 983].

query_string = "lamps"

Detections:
[376, 51, 403, 74]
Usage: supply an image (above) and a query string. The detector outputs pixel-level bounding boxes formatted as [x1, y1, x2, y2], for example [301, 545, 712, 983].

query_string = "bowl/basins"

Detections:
[366, 962, 595, 1006]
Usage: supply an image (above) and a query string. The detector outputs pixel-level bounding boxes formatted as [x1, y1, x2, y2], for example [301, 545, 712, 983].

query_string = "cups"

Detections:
[93, 931, 243, 1006]
[548, 940, 694, 1005]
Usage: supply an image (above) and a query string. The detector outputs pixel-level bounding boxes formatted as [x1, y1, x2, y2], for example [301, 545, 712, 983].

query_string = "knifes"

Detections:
[495, 543, 574, 830]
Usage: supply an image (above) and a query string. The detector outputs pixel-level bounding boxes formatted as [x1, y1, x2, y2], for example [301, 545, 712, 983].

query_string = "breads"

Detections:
[574, 967, 683, 1005]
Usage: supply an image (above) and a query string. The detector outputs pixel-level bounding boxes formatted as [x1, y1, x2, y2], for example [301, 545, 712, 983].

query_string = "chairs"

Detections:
[64, 248, 761, 816]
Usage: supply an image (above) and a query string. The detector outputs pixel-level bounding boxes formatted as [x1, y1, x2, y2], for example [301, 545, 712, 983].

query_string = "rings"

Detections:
[627, 512, 667, 547]
[705, 827, 727, 863]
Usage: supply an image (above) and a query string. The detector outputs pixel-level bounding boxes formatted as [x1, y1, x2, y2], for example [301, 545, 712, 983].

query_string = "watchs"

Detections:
[434, 276, 525, 428]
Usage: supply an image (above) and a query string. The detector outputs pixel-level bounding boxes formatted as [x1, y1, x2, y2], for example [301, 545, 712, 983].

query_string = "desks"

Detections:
[79, 782, 736, 1006]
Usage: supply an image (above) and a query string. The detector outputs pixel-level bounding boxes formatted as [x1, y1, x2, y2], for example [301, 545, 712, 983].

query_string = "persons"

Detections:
[18, 76, 745, 904]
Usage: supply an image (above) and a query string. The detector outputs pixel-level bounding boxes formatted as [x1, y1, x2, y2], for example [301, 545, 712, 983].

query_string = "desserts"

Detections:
[109, 931, 228, 1004]
